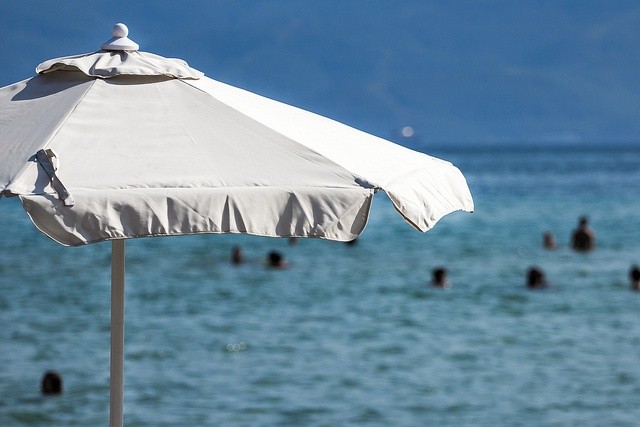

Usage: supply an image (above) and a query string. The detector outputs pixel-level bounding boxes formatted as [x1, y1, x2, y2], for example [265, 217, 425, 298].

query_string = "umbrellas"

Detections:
[0, 21, 476, 427]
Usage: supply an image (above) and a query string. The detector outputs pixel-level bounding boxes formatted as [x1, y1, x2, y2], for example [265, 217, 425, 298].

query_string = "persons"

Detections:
[432, 268, 445, 286]
[526, 267, 544, 289]
[233, 246, 242, 264]
[572, 216, 594, 251]
[266, 251, 281, 267]
[41, 369, 61, 396]
[628, 264, 640, 293]
[543, 231, 553, 251]
